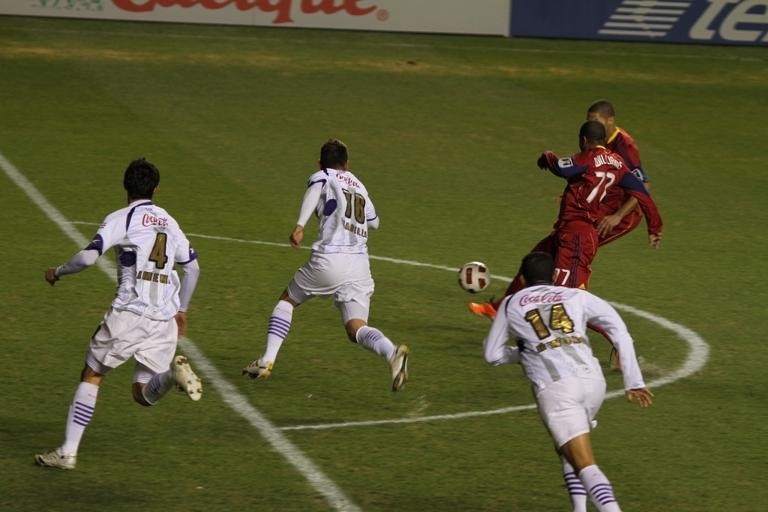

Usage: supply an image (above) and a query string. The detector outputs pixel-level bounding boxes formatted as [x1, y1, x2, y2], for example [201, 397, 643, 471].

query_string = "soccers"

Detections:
[458, 262, 491, 292]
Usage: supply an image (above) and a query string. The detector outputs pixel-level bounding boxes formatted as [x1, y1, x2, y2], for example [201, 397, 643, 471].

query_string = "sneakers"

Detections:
[611, 349, 623, 374]
[386, 342, 412, 394]
[240, 357, 276, 380]
[169, 353, 205, 402]
[33, 447, 79, 472]
[467, 298, 498, 323]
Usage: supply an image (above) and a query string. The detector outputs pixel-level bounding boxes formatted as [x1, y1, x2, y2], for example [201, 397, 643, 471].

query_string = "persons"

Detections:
[241, 139, 410, 393]
[467, 121, 663, 371]
[34, 156, 203, 471]
[482, 251, 654, 512]
[553, 100, 650, 247]
